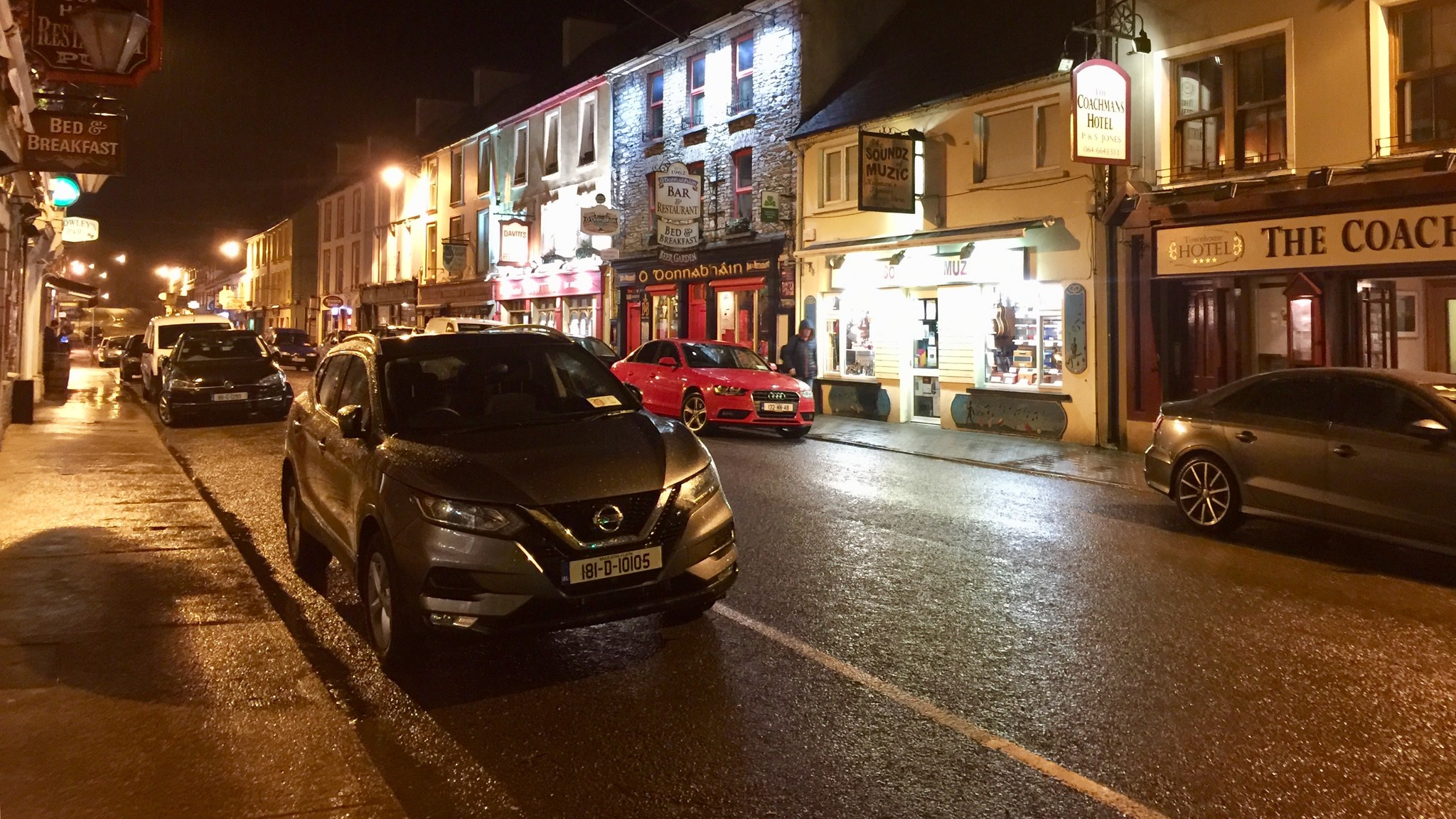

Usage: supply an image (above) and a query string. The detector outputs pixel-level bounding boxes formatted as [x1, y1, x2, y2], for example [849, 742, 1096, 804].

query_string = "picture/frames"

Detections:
[1377, 291, 1419, 339]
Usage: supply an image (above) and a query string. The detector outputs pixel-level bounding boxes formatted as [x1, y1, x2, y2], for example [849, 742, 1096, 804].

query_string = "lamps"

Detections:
[960, 242, 975, 260]
[9, 201, 41, 220]
[1213, 179, 1265, 201]
[833, 254, 846, 270]
[0, 150, 21, 177]
[730, 5, 776, 26]
[1307, 166, 1367, 188]
[1118, 190, 1177, 212]
[888, 248, 907, 265]
[678, 29, 720, 50]
[597, 67, 634, 86]
[898, 129, 926, 142]
[1052, 30, 1078, 74]
[637, 47, 677, 67]
[1123, 12, 1151, 56]
[1424, 151, 1456, 172]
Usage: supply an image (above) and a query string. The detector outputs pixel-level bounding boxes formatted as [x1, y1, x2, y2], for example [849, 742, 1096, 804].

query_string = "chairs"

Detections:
[188, 344, 202, 355]
[384, 360, 422, 395]
[483, 343, 550, 413]
[231, 338, 262, 356]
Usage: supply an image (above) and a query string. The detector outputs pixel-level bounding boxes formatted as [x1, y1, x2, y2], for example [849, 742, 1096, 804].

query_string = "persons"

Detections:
[43, 319, 59, 351]
[784, 320, 819, 394]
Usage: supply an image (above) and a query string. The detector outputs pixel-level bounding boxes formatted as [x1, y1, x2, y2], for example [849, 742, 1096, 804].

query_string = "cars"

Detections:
[157, 329, 294, 429]
[609, 336, 815, 438]
[96, 334, 145, 380]
[1144, 366, 1456, 557]
[538, 330, 623, 367]
[317, 330, 363, 362]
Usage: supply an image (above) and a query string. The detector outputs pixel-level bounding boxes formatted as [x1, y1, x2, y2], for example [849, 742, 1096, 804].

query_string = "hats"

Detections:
[50, 320, 59, 326]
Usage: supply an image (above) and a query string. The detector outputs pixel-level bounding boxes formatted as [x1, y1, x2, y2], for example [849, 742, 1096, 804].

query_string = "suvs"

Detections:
[262, 325, 319, 371]
[280, 324, 743, 674]
[369, 323, 420, 338]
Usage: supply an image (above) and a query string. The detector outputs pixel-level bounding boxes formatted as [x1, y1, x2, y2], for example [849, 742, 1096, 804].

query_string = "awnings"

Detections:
[46, 276, 100, 309]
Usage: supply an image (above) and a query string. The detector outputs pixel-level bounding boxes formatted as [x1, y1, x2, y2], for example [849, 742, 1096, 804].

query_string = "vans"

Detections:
[141, 314, 236, 402]
[423, 316, 510, 336]
